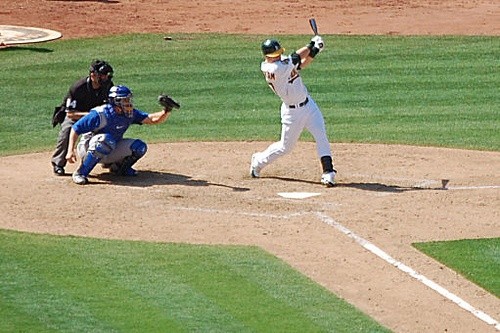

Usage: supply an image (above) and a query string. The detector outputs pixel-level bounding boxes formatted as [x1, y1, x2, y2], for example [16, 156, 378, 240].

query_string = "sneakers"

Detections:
[72, 171, 85, 184]
[115, 168, 137, 176]
[321, 172, 336, 187]
[53, 166, 65, 176]
[250, 154, 260, 178]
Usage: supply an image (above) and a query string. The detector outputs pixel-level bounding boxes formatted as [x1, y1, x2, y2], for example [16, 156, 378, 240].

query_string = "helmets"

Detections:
[109, 85, 132, 99]
[262, 39, 285, 57]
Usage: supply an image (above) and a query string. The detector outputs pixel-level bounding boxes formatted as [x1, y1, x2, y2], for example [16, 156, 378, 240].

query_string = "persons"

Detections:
[250, 35, 337, 187]
[51, 59, 115, 175]
[65, 85, 180, 185]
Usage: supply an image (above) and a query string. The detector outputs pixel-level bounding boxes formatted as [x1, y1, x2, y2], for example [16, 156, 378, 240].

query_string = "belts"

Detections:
[289, 97, 308, 108]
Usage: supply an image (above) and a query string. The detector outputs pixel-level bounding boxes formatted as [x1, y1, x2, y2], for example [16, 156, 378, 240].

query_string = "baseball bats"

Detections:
[308, 18, 323, 48]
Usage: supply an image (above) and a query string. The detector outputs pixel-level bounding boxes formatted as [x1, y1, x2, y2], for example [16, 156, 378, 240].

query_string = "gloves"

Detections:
[306, 36, 324, 58]
[159, 96, 173, 113]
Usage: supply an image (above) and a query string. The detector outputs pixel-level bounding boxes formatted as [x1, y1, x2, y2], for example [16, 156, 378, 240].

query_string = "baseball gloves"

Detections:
[158, 94, 181, 113]
